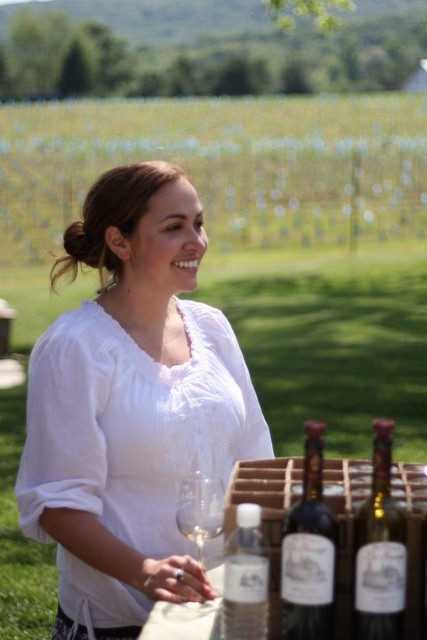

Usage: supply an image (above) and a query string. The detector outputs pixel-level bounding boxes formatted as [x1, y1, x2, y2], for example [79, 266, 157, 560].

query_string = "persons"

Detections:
[12, 160, 276, 640]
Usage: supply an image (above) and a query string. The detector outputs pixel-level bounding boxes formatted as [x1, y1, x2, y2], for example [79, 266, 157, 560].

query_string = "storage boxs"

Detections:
[221, 456, 426, 639]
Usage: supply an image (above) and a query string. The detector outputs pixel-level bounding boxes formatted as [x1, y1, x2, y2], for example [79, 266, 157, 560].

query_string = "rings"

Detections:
[174, 568, 184, 581]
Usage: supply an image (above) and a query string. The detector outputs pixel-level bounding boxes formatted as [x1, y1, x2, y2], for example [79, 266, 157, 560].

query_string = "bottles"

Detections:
[278, 420, 340, 639]
[353, 420, 409, 640]
[221, 503, 270, 640]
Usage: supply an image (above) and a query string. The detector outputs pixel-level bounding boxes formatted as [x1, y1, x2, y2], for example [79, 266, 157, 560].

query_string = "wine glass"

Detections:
[176, 470, 229, 598]
